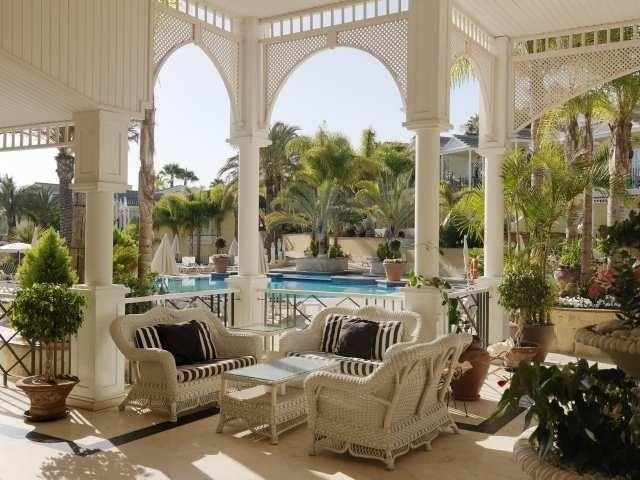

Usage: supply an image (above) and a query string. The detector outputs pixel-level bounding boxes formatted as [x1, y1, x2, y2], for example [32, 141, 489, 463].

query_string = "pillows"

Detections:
[157, 322, 206, 366]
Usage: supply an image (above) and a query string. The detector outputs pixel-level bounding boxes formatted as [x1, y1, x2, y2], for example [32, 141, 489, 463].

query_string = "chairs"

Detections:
[109, 304, 266, 425]
[276, 304, 475, 472]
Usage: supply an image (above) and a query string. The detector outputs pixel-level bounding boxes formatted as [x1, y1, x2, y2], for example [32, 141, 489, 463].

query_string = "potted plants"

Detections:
[381, 241, 408, 281]
[6, 281, 89, 423]
[495, 135, 594, 372]
[211, 237, 231, 274]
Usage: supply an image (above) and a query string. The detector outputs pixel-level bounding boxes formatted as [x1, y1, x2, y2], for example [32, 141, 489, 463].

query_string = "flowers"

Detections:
[588, 249, 640, 330]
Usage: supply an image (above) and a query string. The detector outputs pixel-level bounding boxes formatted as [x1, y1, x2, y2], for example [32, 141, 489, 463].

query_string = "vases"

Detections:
[573, 321, 640, 377]
[451, 332, 492, 404]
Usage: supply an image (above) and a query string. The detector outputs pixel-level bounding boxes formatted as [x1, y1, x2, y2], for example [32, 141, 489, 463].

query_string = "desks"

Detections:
[226, 316, 307, 352]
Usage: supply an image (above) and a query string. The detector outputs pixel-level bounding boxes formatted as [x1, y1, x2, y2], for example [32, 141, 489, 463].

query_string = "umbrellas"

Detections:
[514, 235, 525, 256]
[463, 235, 469, 286]
[150, 233, 180, 287]
[228, 237, 238, 255]
[0, 241, 32, 265]
